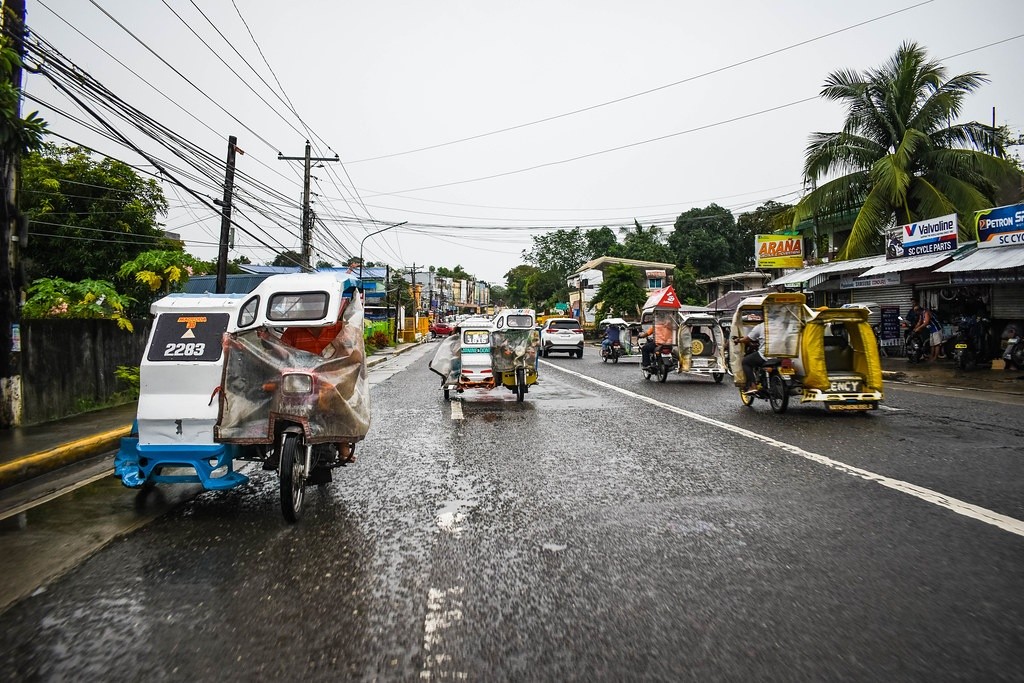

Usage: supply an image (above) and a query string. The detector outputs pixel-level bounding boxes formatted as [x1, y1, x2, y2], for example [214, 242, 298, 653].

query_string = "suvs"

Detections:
[539, 316, 587, 359]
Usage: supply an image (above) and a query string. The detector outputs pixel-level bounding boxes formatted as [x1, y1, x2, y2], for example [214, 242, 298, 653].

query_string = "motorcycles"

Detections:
[636, 285, 727, 384]
[728, 291, 886, 416]
[896, 314, 947, 362]
[950, 312, 993, 373]
[428, 307, 543, 403]
[111, 269, 376, 526]
[598, 317, 629, 365]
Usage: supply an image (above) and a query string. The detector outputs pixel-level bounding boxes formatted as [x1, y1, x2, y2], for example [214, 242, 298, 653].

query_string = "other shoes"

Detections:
[338, 453, 355, 463]
[743, 383, 759, 395]
[263, 454, 279, 469]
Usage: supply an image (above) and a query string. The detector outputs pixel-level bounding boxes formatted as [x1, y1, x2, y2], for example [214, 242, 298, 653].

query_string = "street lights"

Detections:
[359, 219, 407, 279]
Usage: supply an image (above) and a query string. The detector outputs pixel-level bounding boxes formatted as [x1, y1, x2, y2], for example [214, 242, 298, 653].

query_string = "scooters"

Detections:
[1002, 329, 1023, 371]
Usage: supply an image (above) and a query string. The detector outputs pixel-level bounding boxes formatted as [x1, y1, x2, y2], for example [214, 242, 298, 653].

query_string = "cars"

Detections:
[429, 310, 493, 339]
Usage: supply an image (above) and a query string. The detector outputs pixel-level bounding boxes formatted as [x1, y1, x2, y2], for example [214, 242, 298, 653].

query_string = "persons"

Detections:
[272, 293, 360, 463]
[906, 297, 928, 338]
[733, 306, 788, 395]
[913, 306, 942, 362]
[503, 316, 541, 360]
[600, 324, 619, 357]
[638, 312, 673, 370]
[450, 342, 461, 375]
